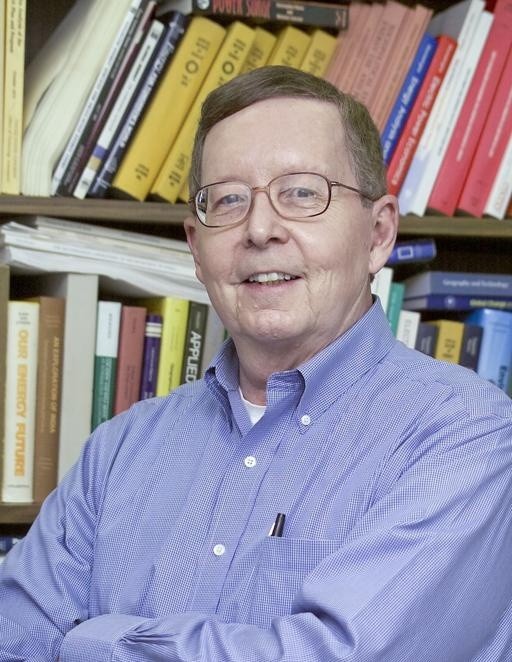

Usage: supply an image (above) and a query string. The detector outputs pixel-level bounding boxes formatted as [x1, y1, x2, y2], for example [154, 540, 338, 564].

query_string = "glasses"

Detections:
[189, 172, 376, 228]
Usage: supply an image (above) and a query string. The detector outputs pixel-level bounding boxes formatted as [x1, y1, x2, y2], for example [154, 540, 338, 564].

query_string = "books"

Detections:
[2, 1, 511, 224]
[374, 244, 512, 395]
[2, 221, 229, 502]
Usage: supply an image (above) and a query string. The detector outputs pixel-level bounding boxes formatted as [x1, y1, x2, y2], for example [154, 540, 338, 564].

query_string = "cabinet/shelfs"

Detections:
[0, 0, 512, 524]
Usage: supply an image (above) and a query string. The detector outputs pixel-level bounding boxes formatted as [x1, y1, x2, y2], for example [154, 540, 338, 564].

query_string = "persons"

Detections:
[1, 67, 512, 662]
